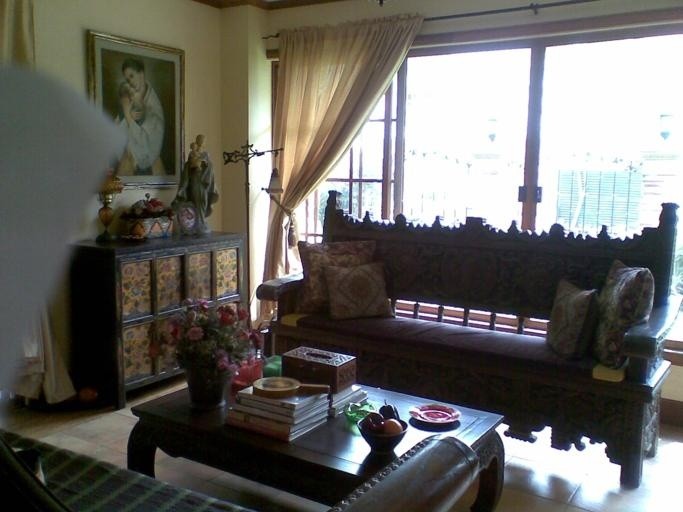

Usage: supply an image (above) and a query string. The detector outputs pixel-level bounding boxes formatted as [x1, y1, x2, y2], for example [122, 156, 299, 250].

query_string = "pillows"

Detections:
[547, 277, 600, 361]
[298, 240, 377, 313]
[322, 259, 396, 320]
[590, 259, 654, 368]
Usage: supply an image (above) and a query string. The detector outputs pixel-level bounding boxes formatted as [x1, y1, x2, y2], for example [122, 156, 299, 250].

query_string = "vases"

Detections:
[120, 212, 174, 242]
[186, 376, 223, 407]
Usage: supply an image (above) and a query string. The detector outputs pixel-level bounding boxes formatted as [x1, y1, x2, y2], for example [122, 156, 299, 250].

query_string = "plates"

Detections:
[407, 402, 462, 427]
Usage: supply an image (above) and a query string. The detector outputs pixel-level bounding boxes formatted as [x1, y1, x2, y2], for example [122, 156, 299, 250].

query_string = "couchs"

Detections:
[1, 431, 481, 512]
[256, 190, 683, 490]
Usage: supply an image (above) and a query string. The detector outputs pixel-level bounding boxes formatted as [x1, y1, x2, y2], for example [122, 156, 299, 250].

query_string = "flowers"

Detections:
[127, 193, 173, 217]
[142, 296, 273, 390]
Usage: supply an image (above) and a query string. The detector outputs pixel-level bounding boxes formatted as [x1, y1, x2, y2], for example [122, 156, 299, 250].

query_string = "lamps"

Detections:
[94, 174, 124, 243]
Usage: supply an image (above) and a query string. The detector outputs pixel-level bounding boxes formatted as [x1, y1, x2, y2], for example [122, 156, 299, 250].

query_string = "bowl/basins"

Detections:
[355, 416, 409, 455]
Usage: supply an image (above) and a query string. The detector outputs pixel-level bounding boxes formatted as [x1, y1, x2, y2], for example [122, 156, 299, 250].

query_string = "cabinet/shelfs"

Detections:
[73, 231, 249, 409]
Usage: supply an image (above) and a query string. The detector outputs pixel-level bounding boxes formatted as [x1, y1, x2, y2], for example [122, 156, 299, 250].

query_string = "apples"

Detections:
[146, 198, 162, 212]
[365, 402, 404, 434]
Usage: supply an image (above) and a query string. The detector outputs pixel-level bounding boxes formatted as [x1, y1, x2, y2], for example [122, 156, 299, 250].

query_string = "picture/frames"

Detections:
[86, 29, 187, 191]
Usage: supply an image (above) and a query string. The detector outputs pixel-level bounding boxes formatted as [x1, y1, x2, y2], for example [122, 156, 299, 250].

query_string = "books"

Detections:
[223, 378, 368, 443]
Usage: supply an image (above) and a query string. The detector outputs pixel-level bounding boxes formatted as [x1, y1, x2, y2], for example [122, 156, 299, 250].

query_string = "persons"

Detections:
[111, 81, 146, 176]
[170, 134, 220, 234]
[108, 57, 168, 175]
